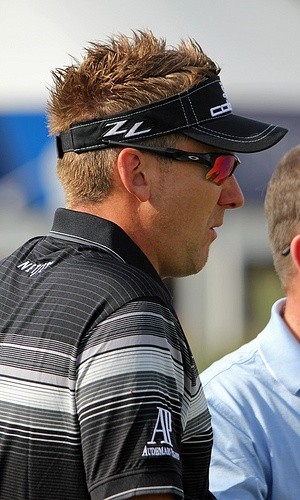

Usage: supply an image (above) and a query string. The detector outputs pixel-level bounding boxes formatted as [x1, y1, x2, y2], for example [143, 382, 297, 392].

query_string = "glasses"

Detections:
[99, 136, 242, 185]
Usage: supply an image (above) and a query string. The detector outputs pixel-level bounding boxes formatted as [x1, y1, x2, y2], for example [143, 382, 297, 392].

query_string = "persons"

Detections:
[197, 145, 299, 499]
[0, 27, 288, 500]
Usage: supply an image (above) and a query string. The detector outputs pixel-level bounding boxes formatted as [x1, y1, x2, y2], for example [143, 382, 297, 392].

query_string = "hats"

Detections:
[53, 73, 289, 159]
